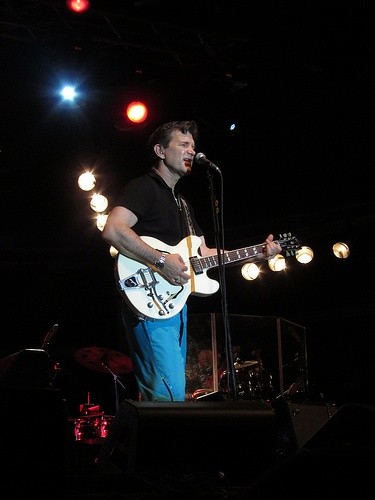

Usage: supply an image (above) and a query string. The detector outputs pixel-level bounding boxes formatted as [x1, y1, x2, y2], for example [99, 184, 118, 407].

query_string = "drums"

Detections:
[190, 389, 213, 399]
[74, 415, 114, 444]
[237, 370, 272, 396]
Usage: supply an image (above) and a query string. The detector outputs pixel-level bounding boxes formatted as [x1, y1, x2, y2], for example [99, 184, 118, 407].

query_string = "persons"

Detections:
[101, 120, 282, 401]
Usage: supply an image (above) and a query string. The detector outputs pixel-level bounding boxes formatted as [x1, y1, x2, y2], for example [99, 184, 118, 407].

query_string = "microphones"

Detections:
[195, 153, 221, 173]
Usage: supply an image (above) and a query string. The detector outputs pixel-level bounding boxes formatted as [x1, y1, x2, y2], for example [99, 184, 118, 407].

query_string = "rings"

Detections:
[176, 276, 182, 284]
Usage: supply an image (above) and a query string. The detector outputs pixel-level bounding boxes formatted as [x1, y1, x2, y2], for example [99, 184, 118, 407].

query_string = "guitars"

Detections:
[114, 232, 302, 322]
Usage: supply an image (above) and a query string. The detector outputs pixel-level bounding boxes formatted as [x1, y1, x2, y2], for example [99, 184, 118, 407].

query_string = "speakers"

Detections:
[100, 400, 375, 500]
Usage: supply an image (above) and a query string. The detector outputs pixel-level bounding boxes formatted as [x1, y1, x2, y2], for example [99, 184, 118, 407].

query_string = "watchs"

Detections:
[152, 252, 166, 272]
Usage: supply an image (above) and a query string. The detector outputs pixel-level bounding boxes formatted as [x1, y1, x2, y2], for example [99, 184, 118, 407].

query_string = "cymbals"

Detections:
[76, 346, 134, 374]
[233, 361, 258, 369]
